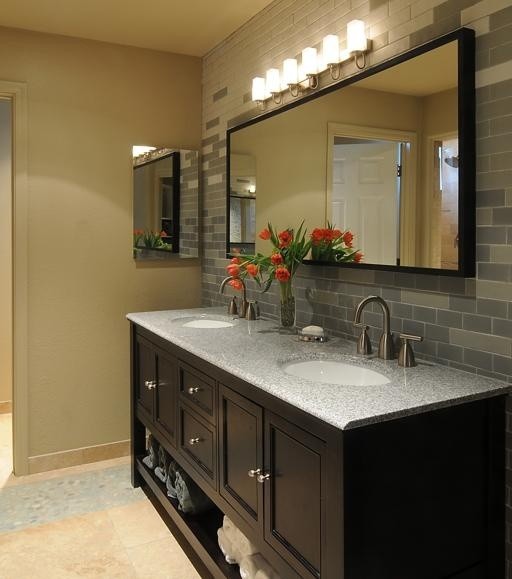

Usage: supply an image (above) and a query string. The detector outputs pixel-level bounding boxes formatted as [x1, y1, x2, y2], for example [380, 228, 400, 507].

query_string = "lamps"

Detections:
[250, 18, 372, 113]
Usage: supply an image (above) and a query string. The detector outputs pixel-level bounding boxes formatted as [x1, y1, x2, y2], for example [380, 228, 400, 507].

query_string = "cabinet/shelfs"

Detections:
[130, 332, 179, 448]
[216, 383, 511, 579]
[179, 368, 218, 491]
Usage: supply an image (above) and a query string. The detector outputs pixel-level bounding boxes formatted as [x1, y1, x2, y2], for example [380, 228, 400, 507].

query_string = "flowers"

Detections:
[226, 216, 313, 300]
[307, 220, 365, 264]
[132, 224, 170, 259]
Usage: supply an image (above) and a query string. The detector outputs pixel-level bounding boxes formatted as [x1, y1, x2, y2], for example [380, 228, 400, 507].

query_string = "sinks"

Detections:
[278, 352, 395, 385]
[173, 314, 239, 329]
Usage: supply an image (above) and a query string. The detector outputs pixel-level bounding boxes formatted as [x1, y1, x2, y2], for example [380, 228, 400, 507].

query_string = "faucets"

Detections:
[219, 275, 247, 318]
[353, 294, 396, 361]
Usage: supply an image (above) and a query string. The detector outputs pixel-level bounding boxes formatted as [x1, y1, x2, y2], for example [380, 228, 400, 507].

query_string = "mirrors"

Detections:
[132, 146, 200, 263]
[225, 25, 477, 279]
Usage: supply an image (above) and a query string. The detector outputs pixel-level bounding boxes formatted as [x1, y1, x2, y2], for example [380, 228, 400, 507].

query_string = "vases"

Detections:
[278, 273, 297, 333]
